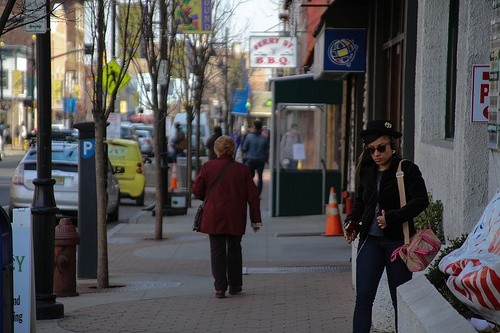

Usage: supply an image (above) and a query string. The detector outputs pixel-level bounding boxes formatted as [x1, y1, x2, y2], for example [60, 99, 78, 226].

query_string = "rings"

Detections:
[344, 233, 348, 236]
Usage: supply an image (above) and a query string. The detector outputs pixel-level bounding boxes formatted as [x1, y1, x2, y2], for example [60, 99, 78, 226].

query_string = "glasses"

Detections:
[366, 140, 391, 153]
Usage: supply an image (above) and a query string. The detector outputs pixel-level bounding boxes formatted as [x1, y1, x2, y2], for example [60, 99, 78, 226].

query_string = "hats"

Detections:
[361, 121, 402, 139]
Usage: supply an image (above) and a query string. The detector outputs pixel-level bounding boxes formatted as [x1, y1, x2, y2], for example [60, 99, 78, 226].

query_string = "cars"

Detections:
[32, 110, 210, 158]
[107, 138, 145, 206]
[9, 141, 125, 224]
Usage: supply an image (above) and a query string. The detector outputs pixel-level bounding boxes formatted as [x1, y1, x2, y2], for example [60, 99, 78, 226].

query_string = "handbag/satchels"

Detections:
[191, 205, 203, 231]
[399, 229, 442, 271]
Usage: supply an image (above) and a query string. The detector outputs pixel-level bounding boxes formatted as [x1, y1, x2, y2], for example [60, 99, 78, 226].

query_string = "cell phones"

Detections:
[346, 221, 361, 234]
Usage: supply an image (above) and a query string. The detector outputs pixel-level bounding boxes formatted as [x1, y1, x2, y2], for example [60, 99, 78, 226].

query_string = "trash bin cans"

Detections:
[177, 157, 201, 191]
[0, 205, 14, 333]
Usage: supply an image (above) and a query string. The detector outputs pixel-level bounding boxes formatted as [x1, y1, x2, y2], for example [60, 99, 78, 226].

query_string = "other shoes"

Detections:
[228, 287, 243, 295]
[215, 291, 226, 298]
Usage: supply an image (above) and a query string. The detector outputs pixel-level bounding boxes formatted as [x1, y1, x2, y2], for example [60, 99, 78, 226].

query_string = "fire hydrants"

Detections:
[55, 218, 81, 297]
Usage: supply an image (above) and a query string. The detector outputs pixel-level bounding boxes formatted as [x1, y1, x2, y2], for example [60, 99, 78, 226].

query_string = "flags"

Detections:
[119, 4, 145, 59]
[171, 0, 213, 34]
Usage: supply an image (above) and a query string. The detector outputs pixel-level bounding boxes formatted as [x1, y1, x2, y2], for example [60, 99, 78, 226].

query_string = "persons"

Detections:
[0, 122, 35, 160]
[173, 120, 301, 199]
[342, 122, 431, 333]
[191, 137, 263, 299]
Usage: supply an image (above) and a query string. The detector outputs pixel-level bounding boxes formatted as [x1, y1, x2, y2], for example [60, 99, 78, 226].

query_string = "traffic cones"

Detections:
[322, 187, 344, 237]
[170, 161, 178, 190]
